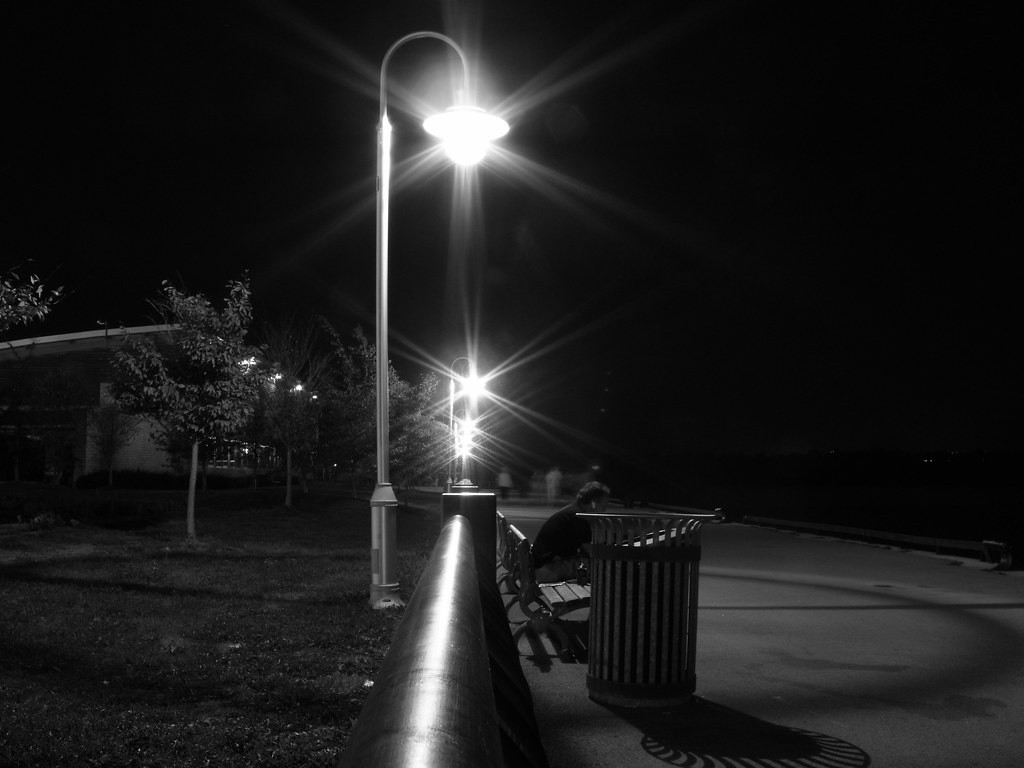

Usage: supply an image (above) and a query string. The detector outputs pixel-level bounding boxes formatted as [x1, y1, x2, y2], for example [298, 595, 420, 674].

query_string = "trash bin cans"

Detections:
[575, 511, 720, 708]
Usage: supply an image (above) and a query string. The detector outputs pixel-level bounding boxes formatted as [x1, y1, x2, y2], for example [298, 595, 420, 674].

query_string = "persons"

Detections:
[530, 481, 610, 583]
[498, 467, 513, 503]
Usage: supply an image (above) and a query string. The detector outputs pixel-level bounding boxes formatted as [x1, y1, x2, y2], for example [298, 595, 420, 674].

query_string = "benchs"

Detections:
[505, 543, 594, 655]
[492, 512, 534, 590]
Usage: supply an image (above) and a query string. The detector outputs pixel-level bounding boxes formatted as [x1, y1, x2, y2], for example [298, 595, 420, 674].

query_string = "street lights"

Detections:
[444, 357, 476, 490]
[364, 27, 512, 612]
